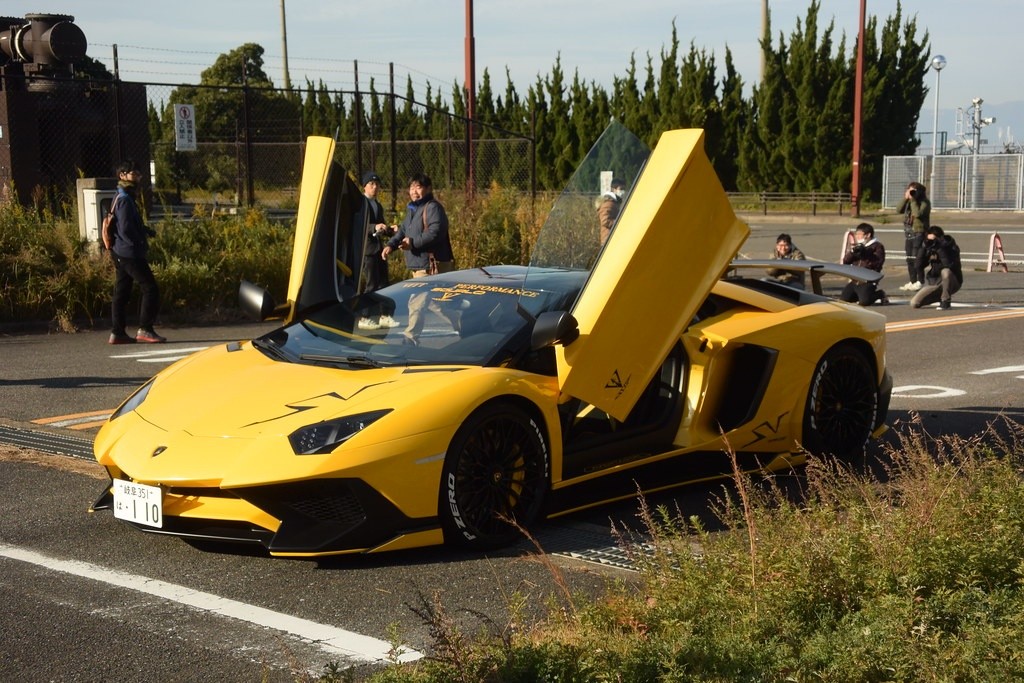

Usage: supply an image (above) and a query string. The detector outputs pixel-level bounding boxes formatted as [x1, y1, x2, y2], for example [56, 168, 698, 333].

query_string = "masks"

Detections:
[614, 189, 625, 198]
[858, 235, 867, 245]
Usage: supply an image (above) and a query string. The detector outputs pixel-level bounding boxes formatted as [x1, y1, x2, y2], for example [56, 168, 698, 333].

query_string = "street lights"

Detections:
[929, 54, 946, 210]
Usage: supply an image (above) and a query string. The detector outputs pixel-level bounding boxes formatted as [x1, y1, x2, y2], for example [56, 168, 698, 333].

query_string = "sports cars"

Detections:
[85, 116, 894, 568]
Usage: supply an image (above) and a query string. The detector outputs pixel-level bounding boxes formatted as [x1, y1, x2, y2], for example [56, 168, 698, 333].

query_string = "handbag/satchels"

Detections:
[102, 213, 116, 249]
[430, 257, 455, 275]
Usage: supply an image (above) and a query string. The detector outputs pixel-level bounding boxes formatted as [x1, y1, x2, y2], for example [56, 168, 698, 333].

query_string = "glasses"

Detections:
[124, 171, 143, 178]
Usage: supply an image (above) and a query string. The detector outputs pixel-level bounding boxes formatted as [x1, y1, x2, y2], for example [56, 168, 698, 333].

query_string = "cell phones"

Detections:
[384, 228, 394, 237]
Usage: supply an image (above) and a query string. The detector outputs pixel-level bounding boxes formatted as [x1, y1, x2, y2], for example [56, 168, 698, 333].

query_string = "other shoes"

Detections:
[879, 289, 889, 305]
[899, 282, 913, 291]
[909, 280, 926, 291]
[936, 302, 951, 310]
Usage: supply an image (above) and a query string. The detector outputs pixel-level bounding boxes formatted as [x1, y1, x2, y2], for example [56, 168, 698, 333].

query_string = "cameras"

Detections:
[924, 238, 939, 249]
[909, 190, 917, 197]
[852, 243, 865, 254]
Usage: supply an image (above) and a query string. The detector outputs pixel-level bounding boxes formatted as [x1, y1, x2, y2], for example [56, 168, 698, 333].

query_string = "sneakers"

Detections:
[358, 318, 381, 330]
[136, 328, 166, 342]
[379, 316, 400, 329]
[109, 333, 137, 344]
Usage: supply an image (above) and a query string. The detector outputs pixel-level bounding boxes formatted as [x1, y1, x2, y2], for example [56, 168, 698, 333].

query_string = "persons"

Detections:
[897, 181, 928, 291]
[911, 226, 963, 311]
[841, 222, 888, 307]
[360, 171, 400, 330]
[102, 157, 166, 344]
[380, 173, 468, 345]
[763, 234, 806, 293]
[595, 178, 625, 248]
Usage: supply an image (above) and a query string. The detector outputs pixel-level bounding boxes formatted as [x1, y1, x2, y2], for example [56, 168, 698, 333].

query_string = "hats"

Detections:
[363, 172, 380, 187]
